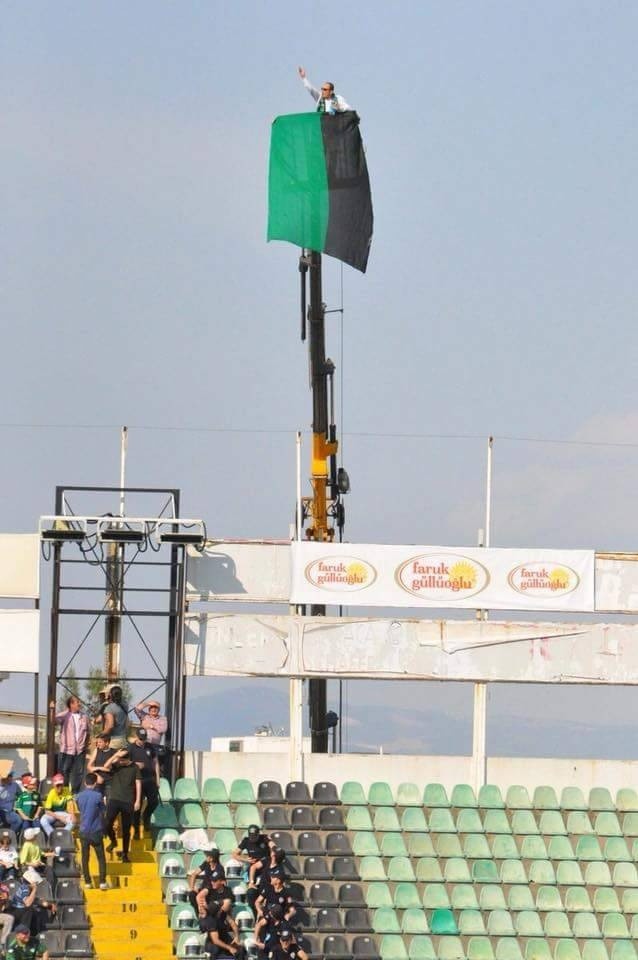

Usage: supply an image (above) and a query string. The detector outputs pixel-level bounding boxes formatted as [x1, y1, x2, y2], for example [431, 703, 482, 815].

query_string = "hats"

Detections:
[22, 775, 38, 786]
[208, 870, 225, 881]
[23, 826, 40, 840]
[52, 773, 64, 785]
[267, 866, 286, 881]
[272, 846, 285, 859]
[136, 727, 147, 740]
[203, 848, 219, 858]
[269, 899, 297, 919]
[279, 925, 293, 941]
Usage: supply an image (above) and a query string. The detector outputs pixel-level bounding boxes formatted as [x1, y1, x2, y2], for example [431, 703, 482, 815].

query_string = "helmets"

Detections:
[248, 825, 260, 842]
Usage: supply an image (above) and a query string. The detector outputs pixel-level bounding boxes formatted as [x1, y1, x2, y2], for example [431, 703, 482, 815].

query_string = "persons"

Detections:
[0, 771, 78, 960]
[187, 824, 308, 960]
[50, 686, 167, 889]
[299, 65, 354, 181]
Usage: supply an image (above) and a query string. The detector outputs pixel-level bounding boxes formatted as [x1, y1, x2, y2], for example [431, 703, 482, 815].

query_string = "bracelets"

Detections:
[150, 724, 153, 729]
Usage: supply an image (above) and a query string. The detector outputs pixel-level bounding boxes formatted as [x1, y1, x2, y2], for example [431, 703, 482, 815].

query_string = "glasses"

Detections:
[321, 87, 332, 91]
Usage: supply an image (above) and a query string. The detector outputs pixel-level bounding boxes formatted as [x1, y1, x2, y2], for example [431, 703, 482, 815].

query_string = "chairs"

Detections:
[150, 778, 638, 959]
[0, 778, 95, 959]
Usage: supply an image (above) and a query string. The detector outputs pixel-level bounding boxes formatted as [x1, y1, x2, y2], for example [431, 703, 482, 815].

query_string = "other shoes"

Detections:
[134, 831, 140, 840]
[142, 814, 151, 832]
[85, 882, 93, 889]
[106, 840, 117, 852]
[122, 852, 128, 863]
[99, 882, 107, 889]
